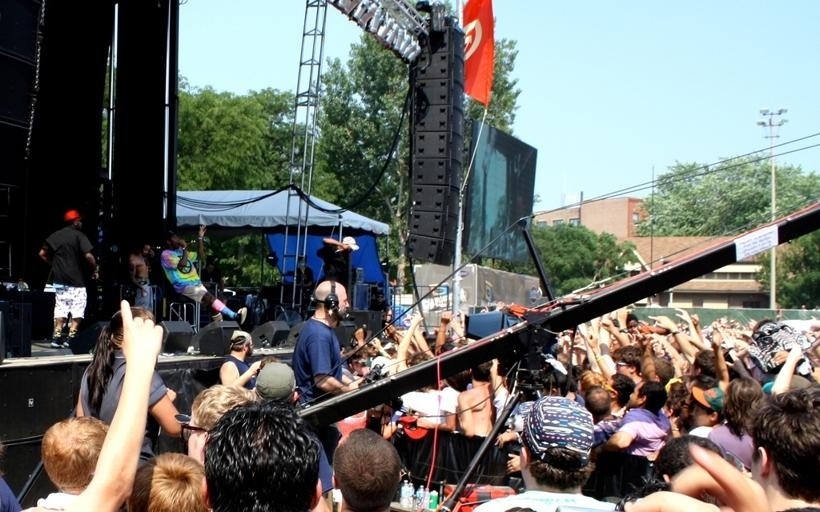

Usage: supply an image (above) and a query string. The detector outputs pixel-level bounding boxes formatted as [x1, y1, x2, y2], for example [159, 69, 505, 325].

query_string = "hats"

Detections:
[255, 362, 296, 405]
[518, 395, 594, 467]
[692, 387, 726, 410]
[343, 236, 359, 251]
[64, 210, 80, 222]
[653, 357, 674, 378]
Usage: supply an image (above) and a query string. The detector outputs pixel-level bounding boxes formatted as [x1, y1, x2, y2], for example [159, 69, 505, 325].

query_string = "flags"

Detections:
[460, 0, 494, 106]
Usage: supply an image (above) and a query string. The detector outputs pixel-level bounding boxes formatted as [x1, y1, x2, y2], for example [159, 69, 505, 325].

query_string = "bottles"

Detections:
[400, 480, 429, 511]
[18, 277, 25, 291]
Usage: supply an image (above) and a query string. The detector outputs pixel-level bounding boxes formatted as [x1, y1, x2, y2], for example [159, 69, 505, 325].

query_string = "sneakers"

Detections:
[51, 339, 63, 347]
[237, 307, 247, 325]
[63, 336, 73, 347]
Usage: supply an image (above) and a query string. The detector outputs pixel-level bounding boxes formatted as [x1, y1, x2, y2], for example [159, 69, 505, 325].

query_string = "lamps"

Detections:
[326, 0, 430, 65]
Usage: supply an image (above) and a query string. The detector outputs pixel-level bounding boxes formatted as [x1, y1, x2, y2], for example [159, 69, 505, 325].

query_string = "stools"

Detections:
[167, 301, 197, 334]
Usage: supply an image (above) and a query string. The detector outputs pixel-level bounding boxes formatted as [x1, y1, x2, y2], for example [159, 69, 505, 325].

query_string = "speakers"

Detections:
[333, 327, 345, 346]
[339, 320, 355, 338]
[405, 27, 464, 266]
[192, 321, 242, 356]
[250, 321, 290, 348]
[158, 321, 195, 353]
[70, 321, 111, 355]
[287, 322, 305, 346]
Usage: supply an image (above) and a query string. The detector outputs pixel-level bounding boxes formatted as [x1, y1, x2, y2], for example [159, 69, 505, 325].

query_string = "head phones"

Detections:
[325, 280, 339, 310]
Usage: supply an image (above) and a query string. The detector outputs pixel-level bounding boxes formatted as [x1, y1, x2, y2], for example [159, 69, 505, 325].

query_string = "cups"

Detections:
[429, 490, 438, 512]
[356, 267, 364, 284]
[187, 345, 194, 354]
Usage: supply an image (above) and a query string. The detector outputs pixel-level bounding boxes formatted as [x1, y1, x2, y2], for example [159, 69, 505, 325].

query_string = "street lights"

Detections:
[757, 105, 789, 309]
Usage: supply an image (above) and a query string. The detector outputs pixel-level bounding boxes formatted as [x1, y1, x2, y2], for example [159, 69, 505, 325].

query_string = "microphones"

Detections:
[344, 313, 356, 321]
[336, 248, 345, 254]
[178, 239, 194, 246]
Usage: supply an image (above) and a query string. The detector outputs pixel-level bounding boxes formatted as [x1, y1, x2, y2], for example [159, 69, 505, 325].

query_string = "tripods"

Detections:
[438, 353, 552, 512]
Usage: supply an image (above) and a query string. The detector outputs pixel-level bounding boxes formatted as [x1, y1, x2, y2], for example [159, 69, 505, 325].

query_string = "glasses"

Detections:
[180, 423, 208, 442]
[616, 362, 631, 370]
